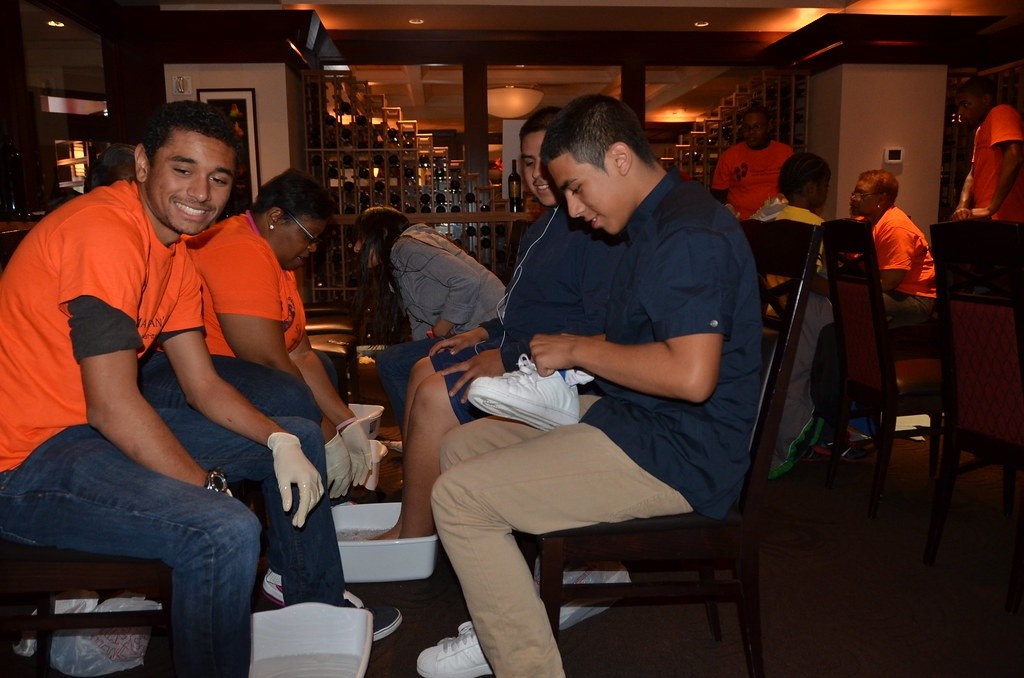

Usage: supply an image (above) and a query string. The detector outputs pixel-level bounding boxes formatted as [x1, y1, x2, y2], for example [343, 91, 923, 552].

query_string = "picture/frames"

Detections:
[196, 87, 262, 223]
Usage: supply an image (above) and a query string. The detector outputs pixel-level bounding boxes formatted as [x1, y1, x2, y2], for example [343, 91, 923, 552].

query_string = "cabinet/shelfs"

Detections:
[301, 67, 510, 326]
[658, 71, 811, 192]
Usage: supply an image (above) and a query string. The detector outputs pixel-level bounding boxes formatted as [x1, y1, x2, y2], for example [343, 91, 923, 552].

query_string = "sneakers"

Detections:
[263, 568, 364, 612]
[360, 603, 401, 641]
[468, 357, 579, 432]
[416, 617, 493, 677]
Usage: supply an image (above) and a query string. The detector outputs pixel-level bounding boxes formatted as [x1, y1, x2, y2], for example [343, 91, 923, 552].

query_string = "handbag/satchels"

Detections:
[50, 592, 162, 676]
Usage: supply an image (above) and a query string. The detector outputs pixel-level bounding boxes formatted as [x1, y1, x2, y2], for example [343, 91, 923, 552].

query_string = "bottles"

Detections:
[313, 221, 521, 289]
[667, 85, 806, 175]
[0, 135, 28, 220]
[508, 159, 522, 213]
[939, 95, 972, 222]
[309, 101, 491, 216]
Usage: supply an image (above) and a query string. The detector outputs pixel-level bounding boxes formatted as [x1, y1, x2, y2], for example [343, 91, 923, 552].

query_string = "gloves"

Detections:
[341, 419, 372, 487]
[323, 433, 351, 498]
[268, 432, 324, 528]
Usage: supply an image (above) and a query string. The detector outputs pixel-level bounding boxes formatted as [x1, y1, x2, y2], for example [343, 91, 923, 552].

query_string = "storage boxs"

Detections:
[347, 403, 385, 440]
[331, 502, 440, 583]
[249, 601, 374, 678]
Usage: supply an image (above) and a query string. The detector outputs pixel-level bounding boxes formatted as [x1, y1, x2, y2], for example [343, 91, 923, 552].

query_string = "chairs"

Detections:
[819, 219, 945, 519]
[304, 313, 363, 335]
[305, 333, 360, 405]
[0, 538, 173, 678]
[536, 216, 821, 678]
[303, 300, 350, 315]
[919, 218, 1024, 620]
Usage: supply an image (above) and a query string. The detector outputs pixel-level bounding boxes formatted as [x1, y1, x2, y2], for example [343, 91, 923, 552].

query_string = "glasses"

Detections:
[286, 212, 318, 246]
[742, 120, 769, 132]
[852, 190, 884, 199]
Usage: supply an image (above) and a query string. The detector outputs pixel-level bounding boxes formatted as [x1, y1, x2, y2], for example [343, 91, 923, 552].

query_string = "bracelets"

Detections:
[336, 417, 357, 430]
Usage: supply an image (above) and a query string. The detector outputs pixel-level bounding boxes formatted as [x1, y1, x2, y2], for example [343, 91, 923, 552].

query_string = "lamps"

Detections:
[487, 82, 546, 119]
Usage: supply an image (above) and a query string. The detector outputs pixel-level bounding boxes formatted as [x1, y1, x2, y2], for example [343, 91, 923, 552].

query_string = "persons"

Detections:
[709, 103, 795, 223]
[758, 149, 938, 472]
[341, 93, 769, 677]
[0, 102, 410, 678]
[945, 72, 1024, 224]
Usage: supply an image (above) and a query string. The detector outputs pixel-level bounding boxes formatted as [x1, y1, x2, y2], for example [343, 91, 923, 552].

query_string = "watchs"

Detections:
[204, 463, 230, 494]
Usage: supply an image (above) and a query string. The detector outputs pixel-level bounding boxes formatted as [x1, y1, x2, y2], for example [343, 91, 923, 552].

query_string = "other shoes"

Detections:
[800, 449, 831, 462]
[811, 437, 868, 462]
[389, 438, 402, 453]
